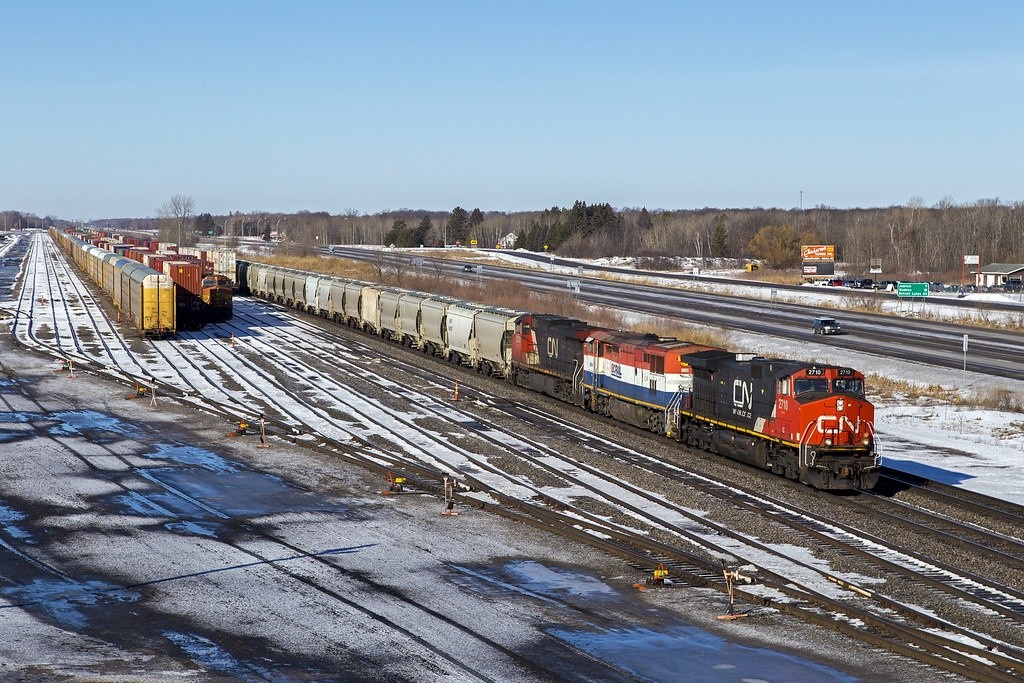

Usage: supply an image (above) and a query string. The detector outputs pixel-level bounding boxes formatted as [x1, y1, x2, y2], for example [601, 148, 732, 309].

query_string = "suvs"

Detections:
[813, 316, 841, 335]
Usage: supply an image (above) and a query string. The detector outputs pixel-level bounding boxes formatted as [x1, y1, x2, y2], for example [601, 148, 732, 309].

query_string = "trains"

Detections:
[83, 227, 884, 490]
[47, 225, 178, 342]
[59, 225, 235, 320]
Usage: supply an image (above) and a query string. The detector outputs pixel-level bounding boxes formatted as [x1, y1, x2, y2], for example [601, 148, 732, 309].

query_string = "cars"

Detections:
[461, 265, 473, 273]
[814, 278, 1024, 294]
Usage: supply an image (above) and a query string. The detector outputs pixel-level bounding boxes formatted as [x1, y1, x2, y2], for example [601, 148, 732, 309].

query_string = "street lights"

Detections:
[241, 217, 251, 235]
[224, 217, 234, 237]
[257, 217, 267, 236]
[276, 217, 287, 234]
